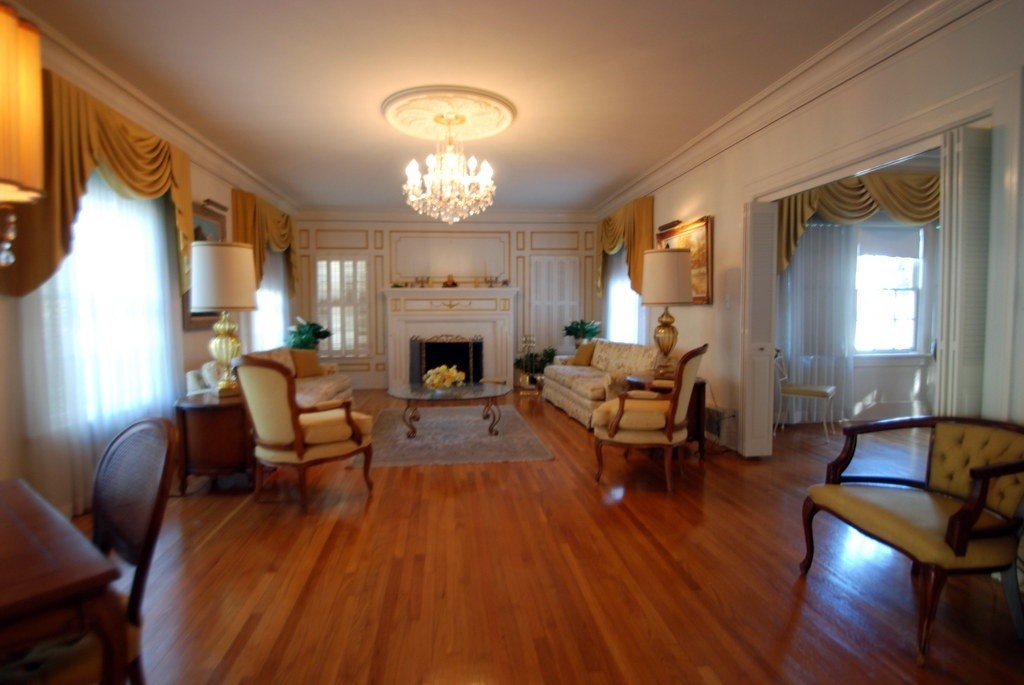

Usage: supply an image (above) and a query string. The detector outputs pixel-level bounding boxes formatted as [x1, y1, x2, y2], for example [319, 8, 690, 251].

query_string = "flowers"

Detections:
[422, 364, 465, 389]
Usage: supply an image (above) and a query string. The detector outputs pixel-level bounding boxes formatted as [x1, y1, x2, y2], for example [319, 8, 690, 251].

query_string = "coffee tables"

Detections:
[387, 382, 511, 438]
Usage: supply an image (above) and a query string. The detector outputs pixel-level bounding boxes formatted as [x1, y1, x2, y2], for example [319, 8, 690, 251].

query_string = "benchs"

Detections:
[800, 415, 1023, 666]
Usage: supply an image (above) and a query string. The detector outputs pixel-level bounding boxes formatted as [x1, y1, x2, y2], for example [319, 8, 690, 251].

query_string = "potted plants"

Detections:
[285, 320, 331, 352]
[514, 353, 544, 397]
[563, 319, 601, 351]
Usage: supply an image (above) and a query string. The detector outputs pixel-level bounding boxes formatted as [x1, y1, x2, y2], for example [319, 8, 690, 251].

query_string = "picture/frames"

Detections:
[183, 200, 227, 328]
[655, 215, 713, 304]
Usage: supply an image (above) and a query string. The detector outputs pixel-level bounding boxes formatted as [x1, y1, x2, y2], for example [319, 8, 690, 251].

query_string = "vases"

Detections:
[436, 388, 451, 393]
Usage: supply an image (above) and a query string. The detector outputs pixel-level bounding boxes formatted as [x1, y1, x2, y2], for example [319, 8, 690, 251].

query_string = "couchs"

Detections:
[543, 337, 653, 431]
[186, 347, 353, 407]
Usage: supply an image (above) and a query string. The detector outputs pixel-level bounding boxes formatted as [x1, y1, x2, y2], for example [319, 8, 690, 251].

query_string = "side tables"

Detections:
[626, 373, 706, 464]
[176, 393, 256, 495]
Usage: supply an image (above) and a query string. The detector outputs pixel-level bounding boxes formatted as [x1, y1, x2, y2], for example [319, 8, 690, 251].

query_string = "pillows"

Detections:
[571, 344, 596, 367]
[290, 349, 323, 376]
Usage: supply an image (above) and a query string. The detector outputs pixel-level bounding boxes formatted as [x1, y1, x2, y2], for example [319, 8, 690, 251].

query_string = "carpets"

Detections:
[346, 404, 555, 468]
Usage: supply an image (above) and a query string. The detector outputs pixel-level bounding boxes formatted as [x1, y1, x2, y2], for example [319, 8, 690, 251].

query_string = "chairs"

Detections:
[773, 351, 836, 443]
[231, 355, 375, 511]
[8, 416, 179, 685]
[591, 342, 708, 492]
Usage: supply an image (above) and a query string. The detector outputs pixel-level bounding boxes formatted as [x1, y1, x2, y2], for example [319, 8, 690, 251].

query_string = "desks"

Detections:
[0, 478, 128, 685]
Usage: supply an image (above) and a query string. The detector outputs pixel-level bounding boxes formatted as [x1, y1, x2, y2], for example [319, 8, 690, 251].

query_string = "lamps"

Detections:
[403, 113, 496, 225]
[0, 4, 44, 268]
[642, 247, 692, 377]
[189, 240, 259, 397]
